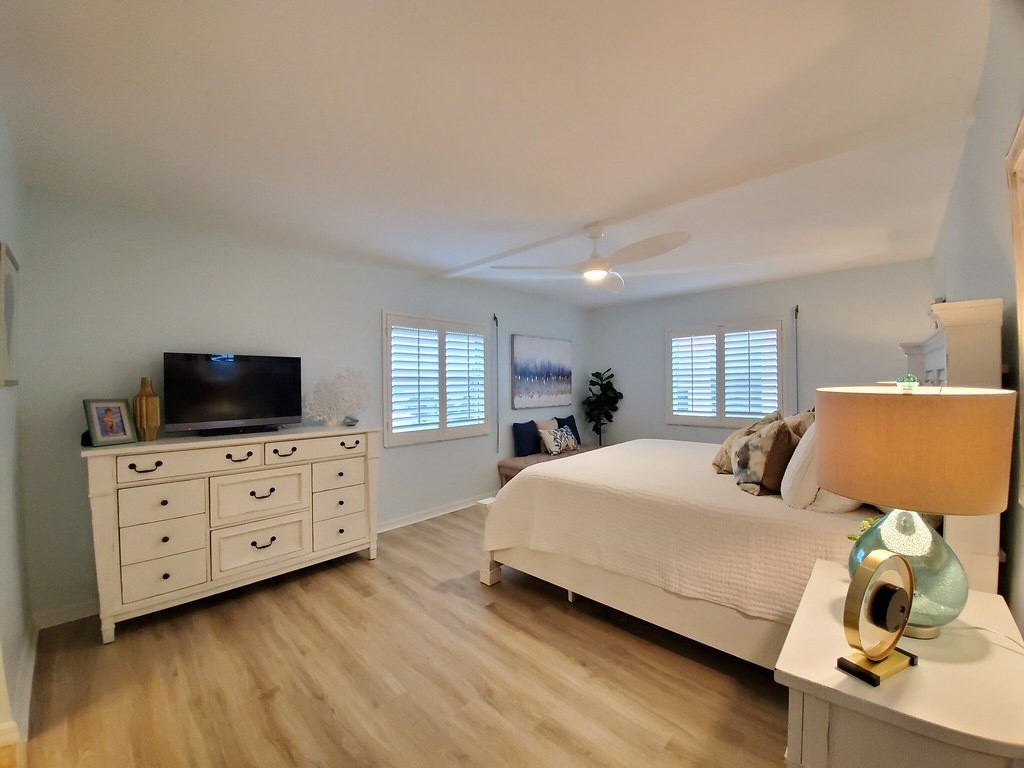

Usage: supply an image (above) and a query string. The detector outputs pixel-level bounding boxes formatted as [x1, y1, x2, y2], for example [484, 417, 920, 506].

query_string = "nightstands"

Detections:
[775, 559, 1024, 768]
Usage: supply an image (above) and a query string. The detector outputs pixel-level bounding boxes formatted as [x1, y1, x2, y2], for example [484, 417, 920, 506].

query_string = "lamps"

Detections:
[813, 385, 1018, 639]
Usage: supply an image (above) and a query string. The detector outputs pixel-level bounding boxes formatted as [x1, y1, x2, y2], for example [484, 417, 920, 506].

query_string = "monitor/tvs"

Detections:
[163, 352, 302, 437]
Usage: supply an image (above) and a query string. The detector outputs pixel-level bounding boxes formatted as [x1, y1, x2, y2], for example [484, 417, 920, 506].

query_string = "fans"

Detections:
[490, 226, 690, 293]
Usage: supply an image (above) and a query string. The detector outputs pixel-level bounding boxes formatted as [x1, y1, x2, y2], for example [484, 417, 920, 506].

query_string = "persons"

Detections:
[105, 409, 119, 434]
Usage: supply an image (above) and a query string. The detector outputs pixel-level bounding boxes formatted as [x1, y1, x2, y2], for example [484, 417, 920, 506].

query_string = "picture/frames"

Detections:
[82, 398, 139, 447]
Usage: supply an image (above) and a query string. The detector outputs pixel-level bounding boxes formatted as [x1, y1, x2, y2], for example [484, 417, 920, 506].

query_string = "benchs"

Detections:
[499, 444, 603, 488]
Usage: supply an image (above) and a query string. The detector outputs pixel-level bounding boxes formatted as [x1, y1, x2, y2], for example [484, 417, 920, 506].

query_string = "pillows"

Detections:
[512, 420, 542, 457]
[712, 407, 864, 513]
[554, 415, 581, 446]
[538, 425, 580, 456]
[535, 419, 559, 453]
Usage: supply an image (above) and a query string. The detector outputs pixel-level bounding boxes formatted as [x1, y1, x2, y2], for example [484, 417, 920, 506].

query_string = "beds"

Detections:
[478, 296, 1011, 672]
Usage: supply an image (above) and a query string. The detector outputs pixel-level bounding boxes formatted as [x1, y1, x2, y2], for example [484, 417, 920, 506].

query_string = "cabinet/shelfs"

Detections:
[79, 429, 380, 645]
